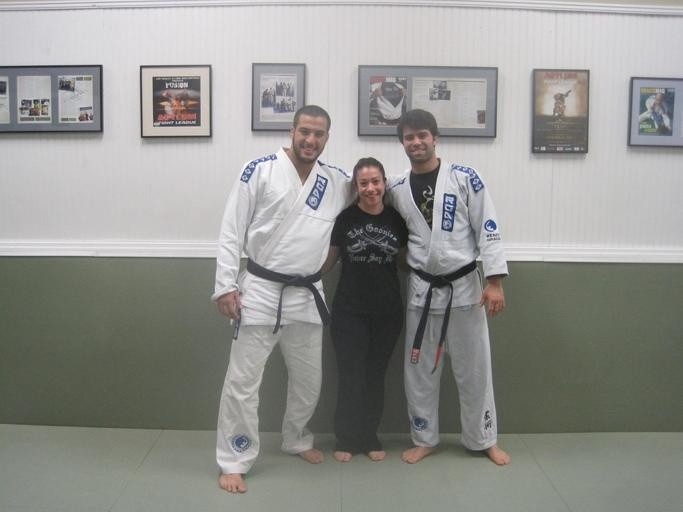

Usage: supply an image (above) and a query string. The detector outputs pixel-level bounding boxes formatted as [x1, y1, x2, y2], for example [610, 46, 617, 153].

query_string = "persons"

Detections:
[638, 92, 671, 136]
[213, 105, 359, 491]
[381, 109, 511, 466]
[319, 156, 408, 463]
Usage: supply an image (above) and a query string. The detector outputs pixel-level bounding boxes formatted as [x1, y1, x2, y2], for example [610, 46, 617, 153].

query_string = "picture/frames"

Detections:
[531, 69, 589, 154]
[139, 65, 211, 138]
[357, 65, 498, 138]
[251, 63, 305, 131]
[627, 77, 683, 147]
[0, 65, 102, 133]
